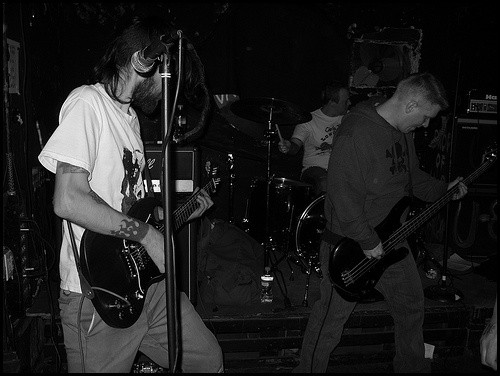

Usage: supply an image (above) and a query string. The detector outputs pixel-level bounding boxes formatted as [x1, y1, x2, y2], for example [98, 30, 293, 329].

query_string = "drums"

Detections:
[243, 178, 315, 253]
[296, 194, 327, 277]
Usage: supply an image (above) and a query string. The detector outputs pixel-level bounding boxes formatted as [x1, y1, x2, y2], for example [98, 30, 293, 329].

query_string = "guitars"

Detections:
[80, 161, 222, 330]
[329, 141, 498, 304]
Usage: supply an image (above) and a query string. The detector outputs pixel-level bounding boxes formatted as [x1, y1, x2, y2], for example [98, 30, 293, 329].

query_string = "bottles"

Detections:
[174, 105, 186, 147]
[134, 362, 163, 373]
[260, 266, 273, 304]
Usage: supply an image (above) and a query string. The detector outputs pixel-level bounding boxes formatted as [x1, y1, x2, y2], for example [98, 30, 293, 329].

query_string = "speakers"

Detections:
[149, 196, 199, 308]
[442, 117, 500, 284]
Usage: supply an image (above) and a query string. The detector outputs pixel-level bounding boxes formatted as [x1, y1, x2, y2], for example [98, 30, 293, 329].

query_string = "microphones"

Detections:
[131, 30, 182, 73]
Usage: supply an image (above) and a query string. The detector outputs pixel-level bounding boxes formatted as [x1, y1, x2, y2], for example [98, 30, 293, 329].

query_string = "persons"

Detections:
[293, 76, 468, 372]
[479, 294, 497, 370]
[277, 82, 352, 194]
[38, 23, 223, 372]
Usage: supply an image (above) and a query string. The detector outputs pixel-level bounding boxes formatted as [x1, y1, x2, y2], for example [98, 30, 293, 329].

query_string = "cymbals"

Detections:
[231, 97, 313, 125]
